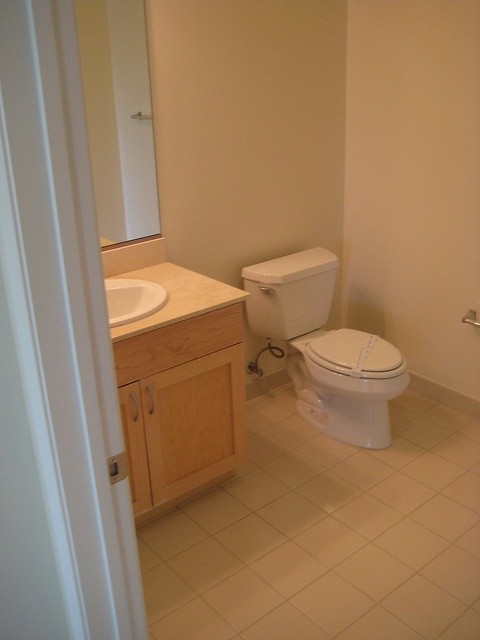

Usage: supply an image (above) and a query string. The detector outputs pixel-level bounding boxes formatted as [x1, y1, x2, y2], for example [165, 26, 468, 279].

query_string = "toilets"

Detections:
[241, 246, 410, 451]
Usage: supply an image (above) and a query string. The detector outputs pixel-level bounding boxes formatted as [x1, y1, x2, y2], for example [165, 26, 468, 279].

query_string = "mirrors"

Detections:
[75, 1, 162, 252]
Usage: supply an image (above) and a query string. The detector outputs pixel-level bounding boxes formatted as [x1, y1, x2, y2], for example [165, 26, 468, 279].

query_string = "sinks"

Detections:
[104, 278, 167, 326]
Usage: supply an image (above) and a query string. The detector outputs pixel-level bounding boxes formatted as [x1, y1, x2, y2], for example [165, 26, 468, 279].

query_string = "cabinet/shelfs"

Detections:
[112, 301, 248, 536]
